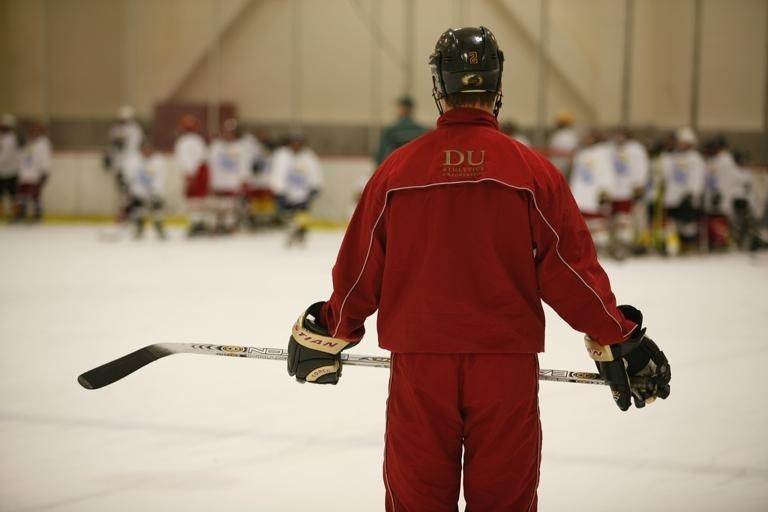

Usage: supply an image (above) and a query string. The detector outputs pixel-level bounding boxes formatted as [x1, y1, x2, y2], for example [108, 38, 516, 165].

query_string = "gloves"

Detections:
[288, 302, 359, 385]
[584, 304, 671, 410]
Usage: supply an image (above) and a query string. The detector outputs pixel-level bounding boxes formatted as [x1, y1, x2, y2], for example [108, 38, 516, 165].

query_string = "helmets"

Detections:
[430, 24, 504, 118]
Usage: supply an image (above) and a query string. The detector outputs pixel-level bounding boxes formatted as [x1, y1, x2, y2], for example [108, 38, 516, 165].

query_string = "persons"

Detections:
[290, 22, 672, 512]
[501, 109, 768, 261]
[0, 113, 22, 219]
[376, 97, 432, 165]
[12, 121, 56, 219]
[105, 103, 321, 242]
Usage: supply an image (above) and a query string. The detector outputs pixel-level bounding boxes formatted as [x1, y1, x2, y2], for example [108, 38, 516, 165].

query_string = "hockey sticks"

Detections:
[78, 342, 670, 399]
